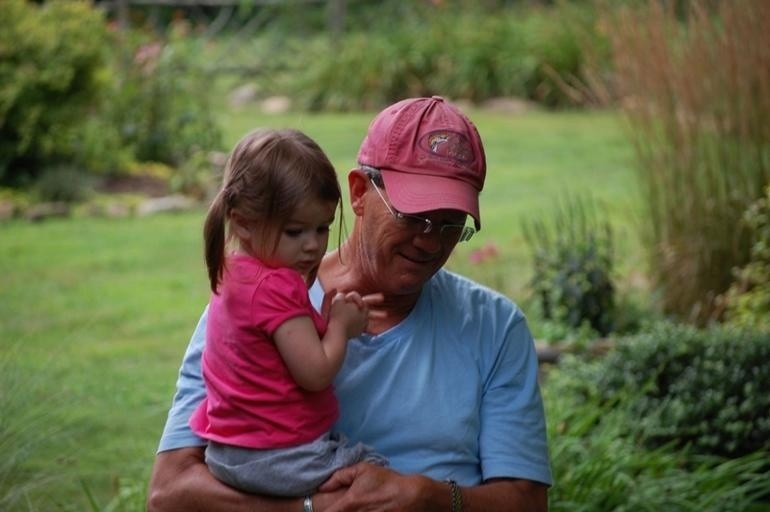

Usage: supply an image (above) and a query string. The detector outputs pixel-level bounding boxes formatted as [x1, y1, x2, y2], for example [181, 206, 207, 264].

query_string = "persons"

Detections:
[200, 127, 393, 497]
[148, 96, 551, 512]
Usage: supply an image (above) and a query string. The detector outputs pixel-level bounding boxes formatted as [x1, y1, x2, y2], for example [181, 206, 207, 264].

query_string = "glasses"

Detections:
[370, 178, 474, 242]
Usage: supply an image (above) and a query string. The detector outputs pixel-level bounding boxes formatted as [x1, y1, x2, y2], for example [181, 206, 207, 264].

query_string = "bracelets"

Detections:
[446, 479, 463, 511]
[304, 492, 313, 511]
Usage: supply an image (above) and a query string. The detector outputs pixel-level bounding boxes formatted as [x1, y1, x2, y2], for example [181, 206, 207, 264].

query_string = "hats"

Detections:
[357, 96, 486, 232]
